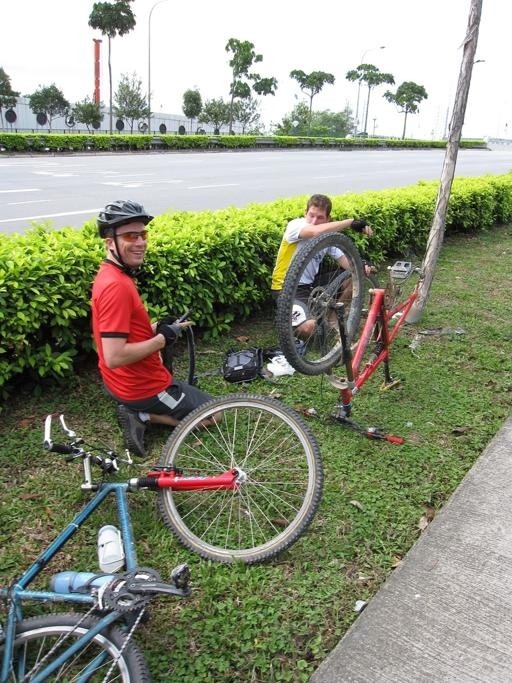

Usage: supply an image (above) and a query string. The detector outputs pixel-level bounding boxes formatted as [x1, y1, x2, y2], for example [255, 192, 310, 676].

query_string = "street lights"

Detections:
[355, 45, 386, 130]
[444, 57, 486, 141]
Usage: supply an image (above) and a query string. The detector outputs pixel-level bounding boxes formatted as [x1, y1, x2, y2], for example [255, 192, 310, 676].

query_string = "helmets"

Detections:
[265, 337, 305, 360]
[97, 200, 155, 238]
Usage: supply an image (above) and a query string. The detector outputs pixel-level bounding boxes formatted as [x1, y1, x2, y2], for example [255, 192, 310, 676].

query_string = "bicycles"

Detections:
[273, 230, 432, 446]
[1, 392, 323, 682]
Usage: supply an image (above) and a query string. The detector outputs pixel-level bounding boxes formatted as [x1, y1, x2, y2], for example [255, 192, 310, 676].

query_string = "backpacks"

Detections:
[222, 346, 263, 384]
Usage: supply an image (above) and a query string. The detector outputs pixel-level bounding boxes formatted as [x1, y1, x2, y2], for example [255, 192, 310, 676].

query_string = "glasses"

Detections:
[116, 230, 148, 242]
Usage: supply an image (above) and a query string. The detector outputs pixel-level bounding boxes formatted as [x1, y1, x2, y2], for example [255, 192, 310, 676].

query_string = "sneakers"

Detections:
[116, 404, 146, 458]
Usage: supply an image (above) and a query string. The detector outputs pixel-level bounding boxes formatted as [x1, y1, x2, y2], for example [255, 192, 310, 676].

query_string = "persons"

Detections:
[92, 200, 225, 458]
[270, 194, 373, 340]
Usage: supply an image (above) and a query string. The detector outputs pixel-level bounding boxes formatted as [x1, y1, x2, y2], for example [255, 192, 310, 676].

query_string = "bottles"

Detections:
[50, 571, 115, 596]
[97, 525, 125, 573]
[366, 342, 385, 366]
